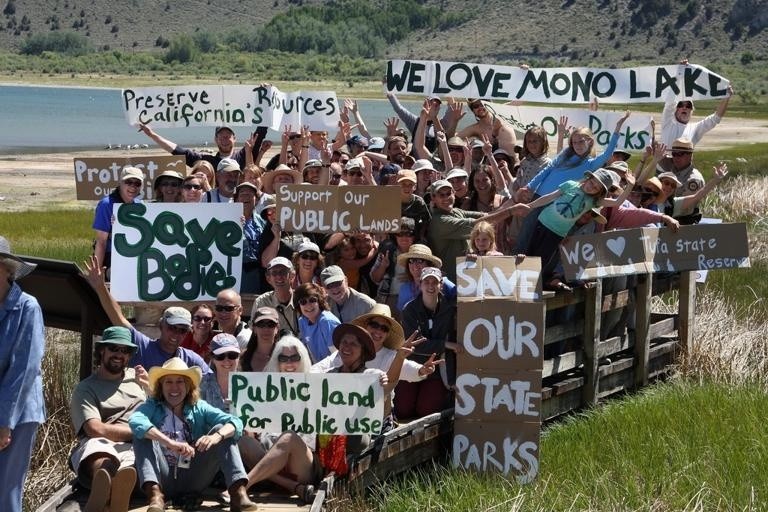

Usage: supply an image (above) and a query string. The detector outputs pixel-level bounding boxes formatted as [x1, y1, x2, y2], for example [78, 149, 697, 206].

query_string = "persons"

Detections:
[466, 222, 526, 262]
[384, 136, 410, 165]
[393, 170, 431, 242]
[180, 304, 217, 363]
[658, 58, 731, 159]
[195, 159, 242, 204]
[467, 165, 509, 243]
[258, 196, 310, 268]
[292, 283, 342, 369]
[344, 98, 383, 148]
[396, 244, 455, 312]
[595, 185, 680, 288]
[154, 170, 185, 203]
[565, 206, 608, 235]
[199, 333, 246, 414]
[91, 167, 145, 281]
[135, 83, 270, 168]
[265, 124, 310, 170]
[370, 218, 414, 314]
[187, 160, 215, 194]
[0, 235, 46, 512]
[127, 357, 258, 512]
[446, 167, 467, 207]
[480, 134, 516, 207]
[651, 138, 705, 225]
[411, 161, 438, 205]
[320, 266, 374, 326]
[237, 334, 387, 504]
[523, 170, 636, 288]
[236, 306, 279, 372]
[292, 242, 324, 297]
[634, 141, 664, 210]
[430, 179, 529, 263]
[394, 268, 461, 420]
[521, 109, 631, 259]
[320, 230, 380, 291]
[312, 303, 445, 432]
[251, 258, 299, 336]
[257, 163, 300, 194]
[182, 175, 203, 203]
[414, 98, 463, 172]
[454, 97, 514, 152]
[69, 327, 151, 512]
[635, 153, 729, 222]
[303, 324, 426, 461]
[599, 161, 629, 191]
[383, 115, 405, 135]
[78, 255, 211, 386]
[325, 227, 380, 296]
[242, 132, 262, 187]
[365, 135, 389, 165]
[303, 122, 352, 157]
[347, 136, 366, 157]
[598, 150, 628, 165]
[228, 180, 267, 292]
[386, 86, 454, 159]
[206, 289, 253, 352]
[373, 162, 397, 184]
[303, 141, 331, 185]
[325, 163, 344, 186]
[347, 156, 378, 185]
[513, 124, 553, 243]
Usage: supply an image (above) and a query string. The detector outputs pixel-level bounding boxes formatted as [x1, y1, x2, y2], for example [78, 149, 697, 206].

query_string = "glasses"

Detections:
[214, 304, 241, 312]
[163, 319, 189, 335]
[121, 179, 142, 188]
[436, 191, 455, 198]
[677, 104, 692, 110]
[215, 134, 233, 141]
[419, 171, 435, 176]
[393, 230, 413, 238]
[494, 157, 506, 163]
[209, 351, 240, 361]
[339, 159, 347, 164]
[525, 138, 541, 145]
[192, 314, 214, 324]
[355, 144, 368, 149]
[286, 161, 298, 168]
[295, 297, 319, 307]
[470, 102, 485, 109]
[296, 251, 319, 262]
[587, 177, 600, 195]
[585, 209, 600, 219]
[369, 149, 383, 153]
[288, 133, 302, 140]
[181, 183, 202, 192]
[671, 151, 690, 158]
[346, 170, 364, 178]
[104, 343, 135, 355]
[325, 279, 346, 290]
[660, 179, 677, 189]
[331, 173, 343, 179]
[473, 149, 486, 155]
[266, 269, 291, 277]
[157, 180, 181, 188]
[366, 320, 391, 334]
[448, 147, 464, 153]
[406, 257, 428, 267]
[568, 138, 591, 146]
[254, 320, 277, 330]
[311, 132, 328, 138]
[277, 352, 302, 364]
[644, 186, 660, 197]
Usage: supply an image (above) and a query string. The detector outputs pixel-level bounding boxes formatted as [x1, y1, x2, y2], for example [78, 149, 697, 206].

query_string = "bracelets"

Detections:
[508, 207, 513, 217]
[216, 431, 224, 441]
[301, 145, 309, 148]
[322, 164, 331, 168]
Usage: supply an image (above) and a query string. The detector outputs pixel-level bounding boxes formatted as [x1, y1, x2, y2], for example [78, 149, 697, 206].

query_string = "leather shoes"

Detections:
[294, 483, 314, 503]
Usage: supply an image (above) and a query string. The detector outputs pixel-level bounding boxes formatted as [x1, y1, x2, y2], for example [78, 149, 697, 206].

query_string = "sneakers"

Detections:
[111, 467, 137, 512]
[86, 468, 111, 512]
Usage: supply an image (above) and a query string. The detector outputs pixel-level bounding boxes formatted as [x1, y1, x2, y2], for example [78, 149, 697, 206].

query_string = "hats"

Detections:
[162, 305, 193, 329]
[583, 167, 614, 197]
[344, 158, 365, 171]
[608, 170, 624, 197]
[208, 331, 242, 358]
[411, 158, 440, 174]
[351, 303, 406, 352]
[319, 264, 348, 290]
[378, 162, 401, 177]
[235, 180, 259, 196]
[396, 169, 418, 185]
[216, 157, 245, 176]
[446, 168, 469, 180]
[419, 267, 444, 285]
[395, 242, 444, 272]
[492, 149, 513, 163]
[339, 153, 349, 161]
[467, 97, 480, 107]
[612, 150, 632, 162]
[604, 160, 630, 174]
[151, 170, 185, 190]
[0, 234, 39, 283]
[470, 139, 485, 149]
[262, 164, 302, 194]
[261, 204, 278, 220]
[644, 175, 666, 205]
[146, 356, 203, 393]
[302, 158, 323, 175]
[294, 242, 321, 258]
[214, 123, 236, 138]
[94, 325, 139, 350]
[589, 206, 608, 226]
[331, 322, 377, 362]
[430, 179, 455, 195]
[266, 256, 294, 272]
[428, 93, 443, 105]
[368, 136, 386, 150]
[665, 138, 696, 154]
[447, 136, 465, 148]
[251, 306, 280, 326]
[349, 135, 369, 148]
[657, 171, 684, 188]
[121, 166, 145, 185]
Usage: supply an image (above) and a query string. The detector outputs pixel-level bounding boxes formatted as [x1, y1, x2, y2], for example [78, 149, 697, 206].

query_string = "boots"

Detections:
[230, 481, 258, 511]
[145, 481, 167, 512]
